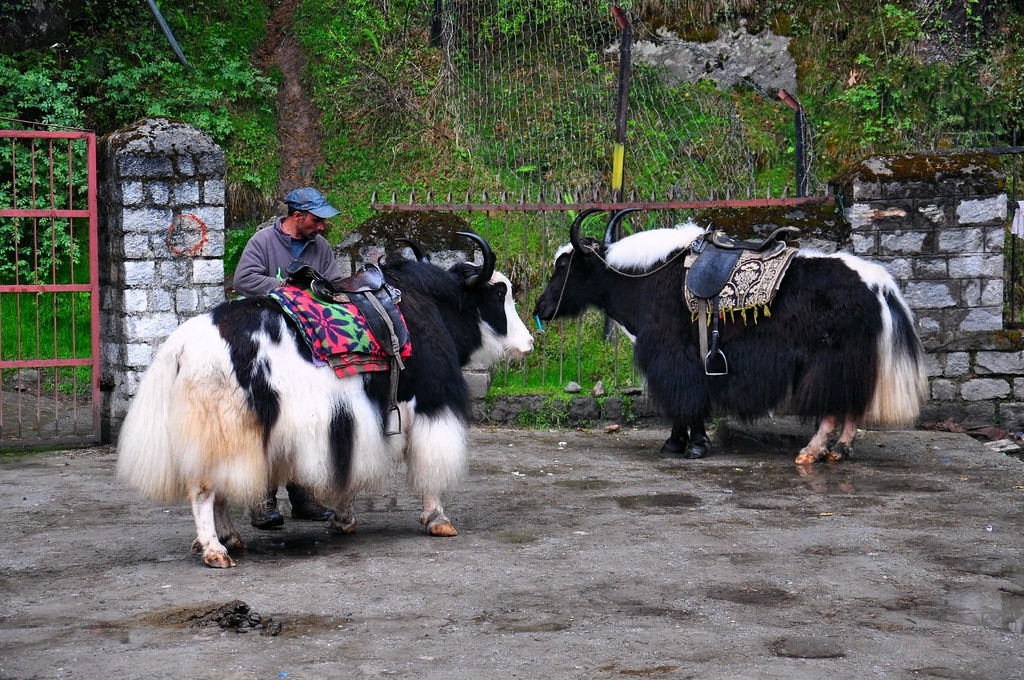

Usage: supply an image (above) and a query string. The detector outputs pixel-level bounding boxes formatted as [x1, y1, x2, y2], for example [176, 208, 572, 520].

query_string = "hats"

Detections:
[283, 187, 341, 218]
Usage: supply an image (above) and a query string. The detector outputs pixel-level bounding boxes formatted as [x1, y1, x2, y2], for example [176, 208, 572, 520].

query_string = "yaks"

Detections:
[534, 210, 925, 463]
[112, 229, 534, 569]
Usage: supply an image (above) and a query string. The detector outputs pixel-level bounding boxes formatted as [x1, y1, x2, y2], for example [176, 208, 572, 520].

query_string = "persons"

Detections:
[234, 189, 342, 527]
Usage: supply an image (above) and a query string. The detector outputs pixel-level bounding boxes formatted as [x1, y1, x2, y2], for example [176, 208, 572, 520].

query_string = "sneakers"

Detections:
[251, 497, 284, 526]
[291, 499, 335, 521]
